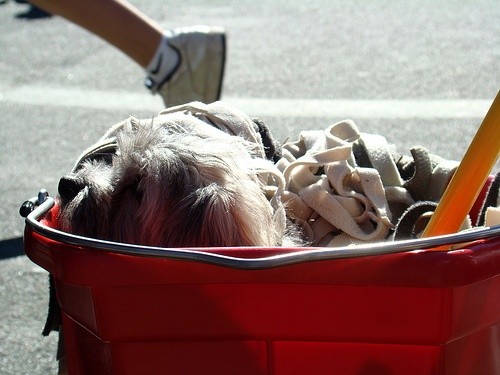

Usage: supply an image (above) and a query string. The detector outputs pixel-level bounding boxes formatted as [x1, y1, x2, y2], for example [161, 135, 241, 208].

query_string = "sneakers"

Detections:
[145, 29, 225, 108]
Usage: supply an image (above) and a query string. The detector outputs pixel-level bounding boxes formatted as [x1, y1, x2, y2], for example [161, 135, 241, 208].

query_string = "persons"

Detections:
[26, 0, 227, 108]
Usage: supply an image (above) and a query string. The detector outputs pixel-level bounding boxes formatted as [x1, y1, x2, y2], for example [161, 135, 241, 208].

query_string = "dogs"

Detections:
[56, 115, 304, 248]
